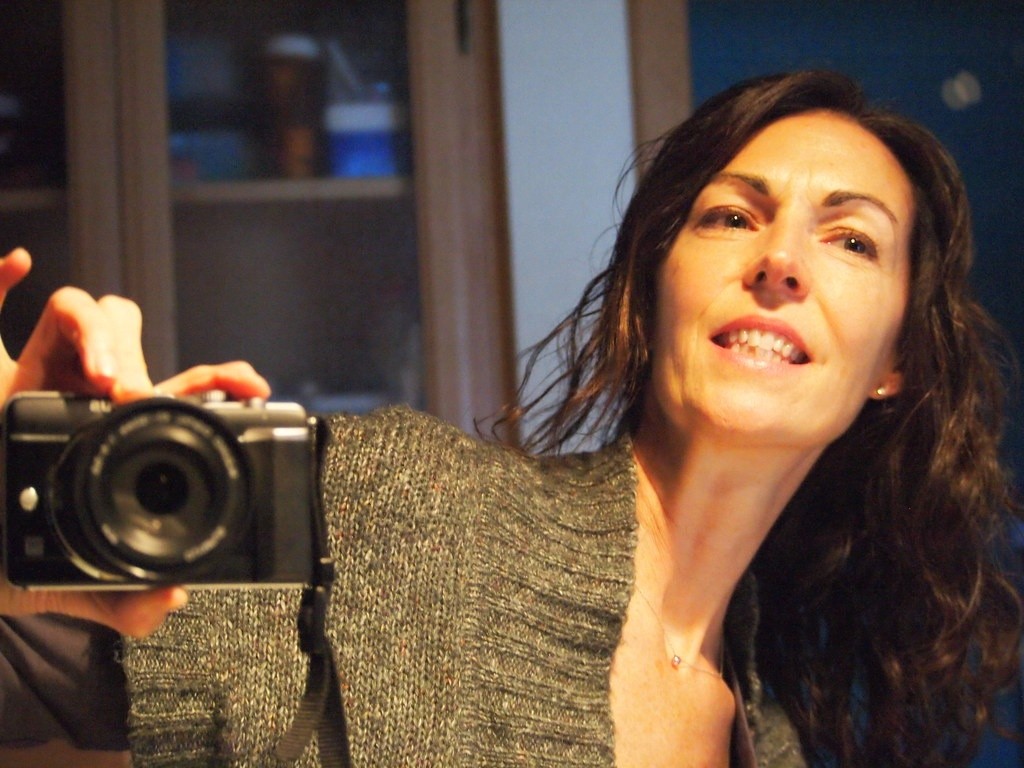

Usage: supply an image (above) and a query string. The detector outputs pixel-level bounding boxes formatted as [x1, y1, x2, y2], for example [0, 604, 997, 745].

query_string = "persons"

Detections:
[0, 65, 1024, 768]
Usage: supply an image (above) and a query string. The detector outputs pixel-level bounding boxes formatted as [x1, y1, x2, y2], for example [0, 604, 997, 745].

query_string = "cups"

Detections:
[324, 101, 413, 179]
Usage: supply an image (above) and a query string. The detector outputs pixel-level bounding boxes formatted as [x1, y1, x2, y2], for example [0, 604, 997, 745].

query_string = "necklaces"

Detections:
[635, 586, 724, 678]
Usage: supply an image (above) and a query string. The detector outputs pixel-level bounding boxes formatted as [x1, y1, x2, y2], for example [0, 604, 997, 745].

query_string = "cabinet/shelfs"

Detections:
[0, 1, 526, 456]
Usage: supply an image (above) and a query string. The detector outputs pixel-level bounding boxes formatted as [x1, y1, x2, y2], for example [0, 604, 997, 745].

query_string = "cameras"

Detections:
[0, 390, 315, 593]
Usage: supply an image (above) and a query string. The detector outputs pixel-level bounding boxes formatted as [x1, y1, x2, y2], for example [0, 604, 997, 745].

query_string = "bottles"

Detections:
[265, 34, 331, 178]
